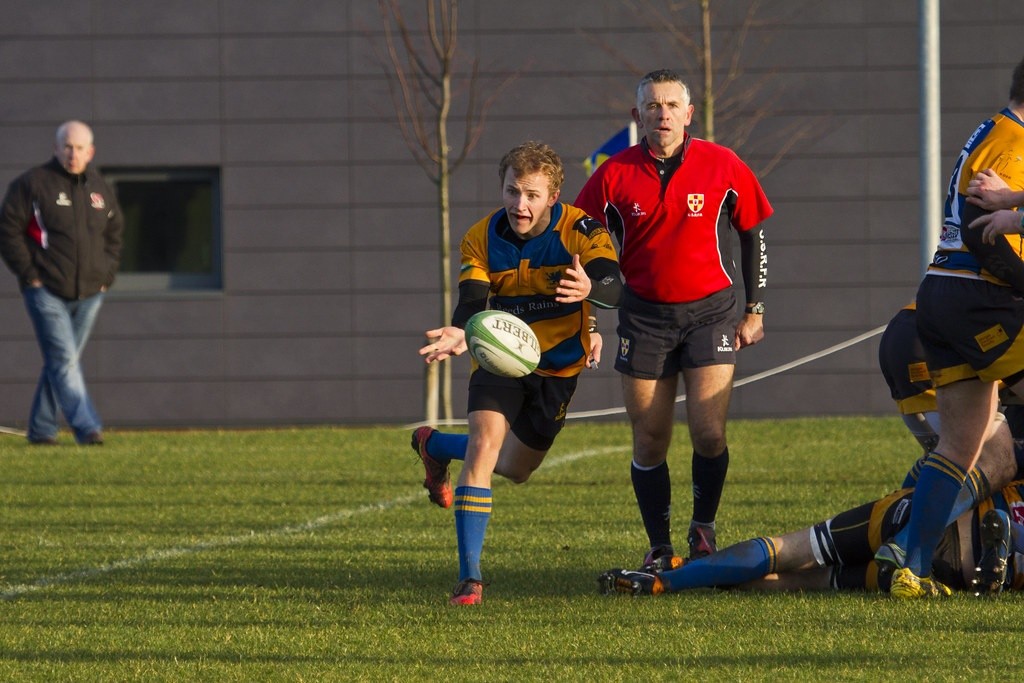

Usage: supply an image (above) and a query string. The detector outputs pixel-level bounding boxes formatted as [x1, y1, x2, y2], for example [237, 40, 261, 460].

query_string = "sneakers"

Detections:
[449, 576, 484, 606]
[411, 427, 454, 509]
[687, 525, 717, 564]
[886, 566, 953, 600]
[645, 554, 695, 575]
[638, 545, 675, 574]
[972, 508, 1013, 597]
[599, 568, 665, 597]
[874, 543, 908, 571]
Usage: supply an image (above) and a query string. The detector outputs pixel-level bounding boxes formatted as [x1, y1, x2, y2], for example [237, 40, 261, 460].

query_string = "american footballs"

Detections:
[465, 310, 541, 378]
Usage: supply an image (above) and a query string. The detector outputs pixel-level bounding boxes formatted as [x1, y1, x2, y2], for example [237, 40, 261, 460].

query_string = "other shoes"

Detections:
[30, 437, 59, 444]
[81, 432, 104, 444]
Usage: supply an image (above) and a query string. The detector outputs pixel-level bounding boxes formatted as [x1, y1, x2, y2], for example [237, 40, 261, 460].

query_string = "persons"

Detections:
[574, 66, 773, 564]
[411, 141, 626, 606]
[0, 121, 123, 446]
[599, 60, 1024, 597]
